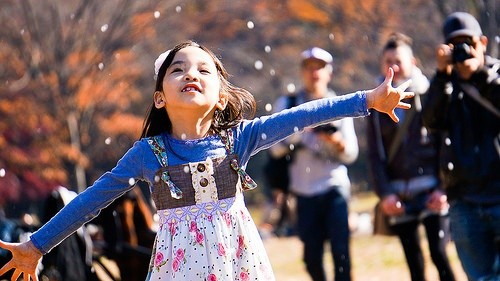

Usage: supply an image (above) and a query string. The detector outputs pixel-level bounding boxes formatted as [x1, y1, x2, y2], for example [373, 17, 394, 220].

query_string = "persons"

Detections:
[0, 177, 160, 281]
[266, 47, 358, 281]
[0, 40, 415, 281]
[420, 9, 500, 281]
[366, 32, 458, 280]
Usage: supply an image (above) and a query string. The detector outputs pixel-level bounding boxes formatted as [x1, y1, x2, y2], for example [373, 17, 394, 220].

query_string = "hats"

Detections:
[300, 47, 333, 65]
[442, 11, 483, 41]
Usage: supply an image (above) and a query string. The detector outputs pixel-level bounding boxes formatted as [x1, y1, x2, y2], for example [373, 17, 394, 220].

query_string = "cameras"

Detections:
[314, 125, 337, 135]
[447, 42, 474, 66]
[404, 189, 432, 215]
